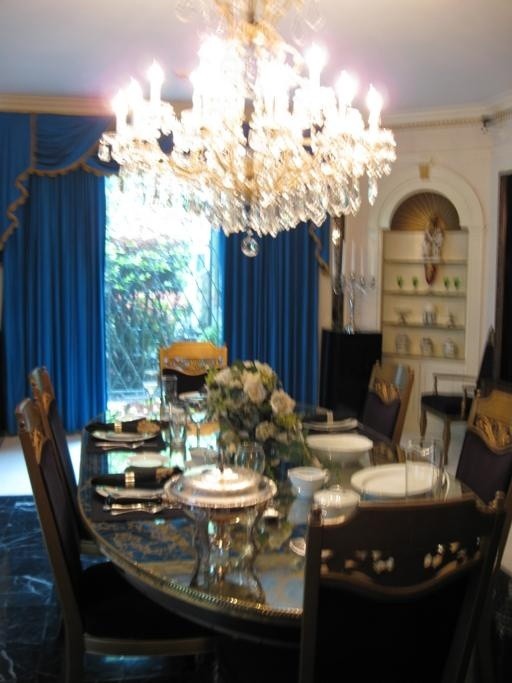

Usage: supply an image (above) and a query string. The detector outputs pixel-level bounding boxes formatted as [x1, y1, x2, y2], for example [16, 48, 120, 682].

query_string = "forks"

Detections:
[93, 441, 145, 449]
[112, 505, 166, 517]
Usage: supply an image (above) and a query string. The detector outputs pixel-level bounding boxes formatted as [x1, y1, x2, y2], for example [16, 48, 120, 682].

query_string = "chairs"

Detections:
[15, 395, 230, 681]
[418, 321, 497, 463]
[453, 386, 512, 587]
[157, 340, 228, 401]
[29, 364, 112, 642]
[365, 363, 415, 446]
[299, 488, 504, 682]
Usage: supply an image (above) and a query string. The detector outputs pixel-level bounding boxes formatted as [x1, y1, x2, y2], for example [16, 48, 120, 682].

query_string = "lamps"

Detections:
[97, 1, 395, 262]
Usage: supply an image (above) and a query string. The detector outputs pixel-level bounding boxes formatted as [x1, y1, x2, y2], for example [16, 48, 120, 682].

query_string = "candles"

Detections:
[358, 245, 365, 274]
[368, 244, 376, 276]
[340, 239, 348, 273]
[349, 239, 356, 272]
[330, 242, 337, 278]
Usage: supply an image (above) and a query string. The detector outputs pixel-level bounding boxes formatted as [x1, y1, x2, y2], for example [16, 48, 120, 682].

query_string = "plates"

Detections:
[348, 461, 442, 498]
[94, 427, 154, 442]
[95, 483, 161, 499]
[308, 432, 372, 451]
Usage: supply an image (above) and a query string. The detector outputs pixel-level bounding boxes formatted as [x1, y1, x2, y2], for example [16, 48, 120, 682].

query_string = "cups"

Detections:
[403, 437, 445, 501]
[159, 375, 220, 470]
[288, 465, 360, 524]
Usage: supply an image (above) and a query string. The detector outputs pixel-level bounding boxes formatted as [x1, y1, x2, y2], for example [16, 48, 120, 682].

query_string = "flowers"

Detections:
[198, 356, 323, 467]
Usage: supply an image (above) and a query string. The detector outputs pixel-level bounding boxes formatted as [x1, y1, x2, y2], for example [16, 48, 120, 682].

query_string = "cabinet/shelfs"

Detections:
[320, 325, 383, 424]
[378, 225, 467, 364]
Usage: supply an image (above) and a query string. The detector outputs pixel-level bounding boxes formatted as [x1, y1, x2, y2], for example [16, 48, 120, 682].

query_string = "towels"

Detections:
[88, 465, 183, 489]
[300, 408, 356, 424]
[83, 417, 169, 436]
